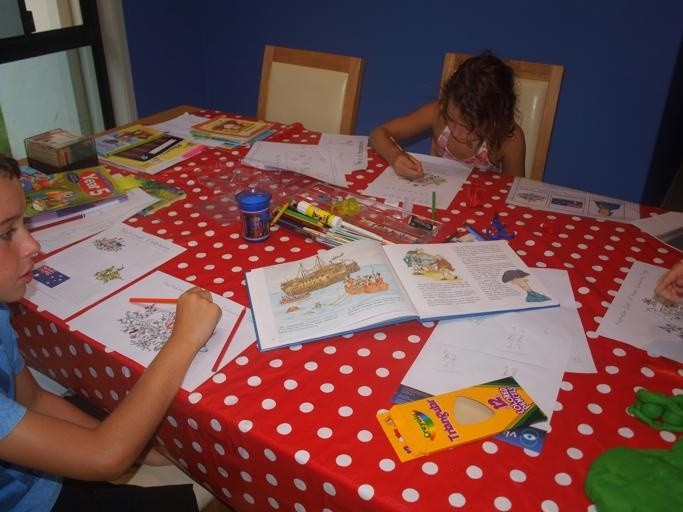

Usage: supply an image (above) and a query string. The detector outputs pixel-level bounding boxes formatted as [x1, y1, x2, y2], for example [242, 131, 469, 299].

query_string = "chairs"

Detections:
[257, 43, 366, 136]
[431, 53, 568, 182]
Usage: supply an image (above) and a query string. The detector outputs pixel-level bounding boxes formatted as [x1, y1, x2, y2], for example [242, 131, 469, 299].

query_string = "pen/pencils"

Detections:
[432, 192, 435, 229]
[389, 136, 416, 163]
[270, 198, 398, 249]
[211, 306, 247, 372]
[462, 224, 487, 241]
[129, 298, 177, 303]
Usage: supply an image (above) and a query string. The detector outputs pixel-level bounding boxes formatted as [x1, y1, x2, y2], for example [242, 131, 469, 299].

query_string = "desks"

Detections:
[12, 104, 683, 512]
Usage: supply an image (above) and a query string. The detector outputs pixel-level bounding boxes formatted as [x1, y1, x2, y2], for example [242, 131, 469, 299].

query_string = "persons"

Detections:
[0, 156, 222, 512]
[655, 258, 683, 304]
[369, 52, 527, 180]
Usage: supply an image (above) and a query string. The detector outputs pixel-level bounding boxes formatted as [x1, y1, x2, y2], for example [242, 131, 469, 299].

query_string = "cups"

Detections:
[234, 186, 273, 243]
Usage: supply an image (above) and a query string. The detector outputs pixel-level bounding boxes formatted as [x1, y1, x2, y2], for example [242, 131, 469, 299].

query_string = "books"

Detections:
[244, 239, 560, 353]
[21, 164, 132, 226]
[190, 115, 277, 147]
[98, 123, 204, 176]
